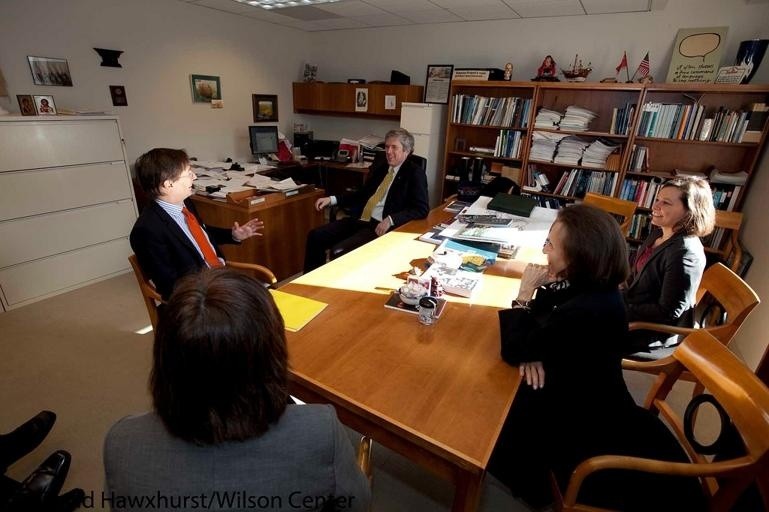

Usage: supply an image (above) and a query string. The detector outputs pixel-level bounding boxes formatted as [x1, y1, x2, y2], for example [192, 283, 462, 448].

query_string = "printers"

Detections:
[300, 138, 340, 160]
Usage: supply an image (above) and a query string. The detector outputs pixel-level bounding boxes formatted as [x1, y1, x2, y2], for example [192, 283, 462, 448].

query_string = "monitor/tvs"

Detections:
[249, 126, 279, 164]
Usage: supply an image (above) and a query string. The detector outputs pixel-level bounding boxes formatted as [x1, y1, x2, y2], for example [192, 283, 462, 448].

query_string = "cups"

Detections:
[418, 296, 438, 325]
[417, 322, 437, 346]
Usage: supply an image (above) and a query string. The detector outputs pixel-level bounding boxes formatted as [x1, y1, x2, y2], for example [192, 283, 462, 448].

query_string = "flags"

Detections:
[637, 53, 650, 77]
[615, 52, 627, 75]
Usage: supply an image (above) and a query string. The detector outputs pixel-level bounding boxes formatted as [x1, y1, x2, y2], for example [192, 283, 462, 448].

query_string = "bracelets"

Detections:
[510, 299, 533, 309]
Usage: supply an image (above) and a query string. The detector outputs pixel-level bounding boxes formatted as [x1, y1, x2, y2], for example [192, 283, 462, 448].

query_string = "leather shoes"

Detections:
[20, 451, 71, 506]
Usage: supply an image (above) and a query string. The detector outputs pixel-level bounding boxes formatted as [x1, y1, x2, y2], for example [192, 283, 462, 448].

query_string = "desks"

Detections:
[190, 157, 376, 279]
[260, 193, 568, 508]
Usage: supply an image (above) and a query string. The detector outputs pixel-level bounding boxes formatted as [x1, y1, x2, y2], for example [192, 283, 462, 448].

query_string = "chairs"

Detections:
[327, 152, 428, 257]
[126, 253, 276, 336]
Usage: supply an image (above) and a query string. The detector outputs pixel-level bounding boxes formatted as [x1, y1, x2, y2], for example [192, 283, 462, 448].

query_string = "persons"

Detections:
[622, 174, 716, 357]
[428, 67, 451, 79]
[302, 128, 430, 276]
[1, 408, 72, 511]
[493, 203, 648, 511]
[20, 98, 32, 114]
[39, 98, 54, 113]
[102, 263, 373, 511]
[129, 147, 265, 305]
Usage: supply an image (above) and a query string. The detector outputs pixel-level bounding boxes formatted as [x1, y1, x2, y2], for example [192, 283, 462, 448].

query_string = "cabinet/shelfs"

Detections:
[293, 82, 424, 117]
[0, 114, 145, 320]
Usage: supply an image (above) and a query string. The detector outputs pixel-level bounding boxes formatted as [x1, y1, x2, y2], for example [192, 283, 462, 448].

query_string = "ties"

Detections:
[182, 208, 224, 269]
[361, 168, 394, 222]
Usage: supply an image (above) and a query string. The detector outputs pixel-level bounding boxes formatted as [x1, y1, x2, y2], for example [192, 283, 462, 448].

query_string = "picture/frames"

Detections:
[189, 72, 222, 105]
[26, 55, 73, 87]
[424, 66, 453, 105]
[16, 93, 58, 116]
[251, 92, 279, 123]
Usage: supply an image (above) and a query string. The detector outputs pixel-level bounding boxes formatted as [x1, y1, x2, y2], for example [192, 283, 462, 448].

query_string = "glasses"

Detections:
[178, 171, 194, 179]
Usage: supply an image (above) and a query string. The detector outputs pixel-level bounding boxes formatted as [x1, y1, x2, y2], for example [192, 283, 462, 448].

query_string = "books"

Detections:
[334, 133, 386, 168]
[265, 288, 329, 334]
[418, 223, 448, 245]
[443, 92, 769, 272]
[57, 104, 112, 115]
[422, 261, 483, 298]
[443, 199, 472, 214]
[185, 157, 307, 201]
[382, 291, 447, 319]
[423, 239, 499, 275]
